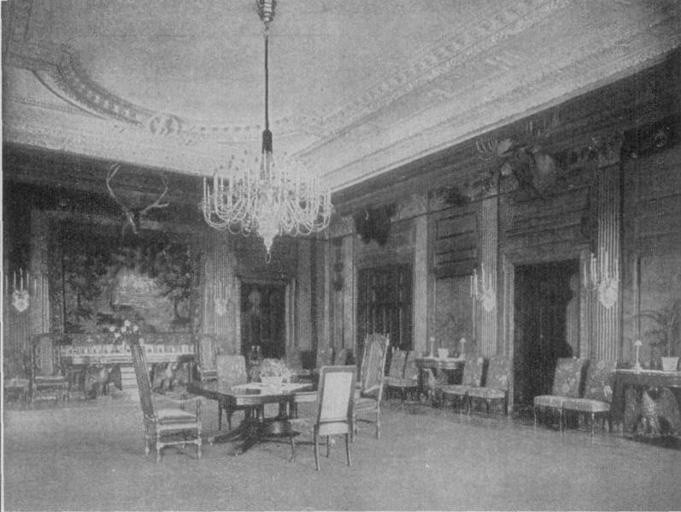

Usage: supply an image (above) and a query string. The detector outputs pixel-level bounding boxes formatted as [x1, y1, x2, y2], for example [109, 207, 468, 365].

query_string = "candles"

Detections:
[4, 268, 39, 300]
[580, 246, 619, 289]
[468, 261, 493, 300]
[209, 280, 234, 299]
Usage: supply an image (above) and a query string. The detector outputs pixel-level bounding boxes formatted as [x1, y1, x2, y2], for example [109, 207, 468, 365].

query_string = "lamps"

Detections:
[193, 2, 338, 260]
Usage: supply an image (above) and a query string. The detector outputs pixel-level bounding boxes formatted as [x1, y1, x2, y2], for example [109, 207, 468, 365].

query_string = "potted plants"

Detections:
[625, 300, 681, 373]
